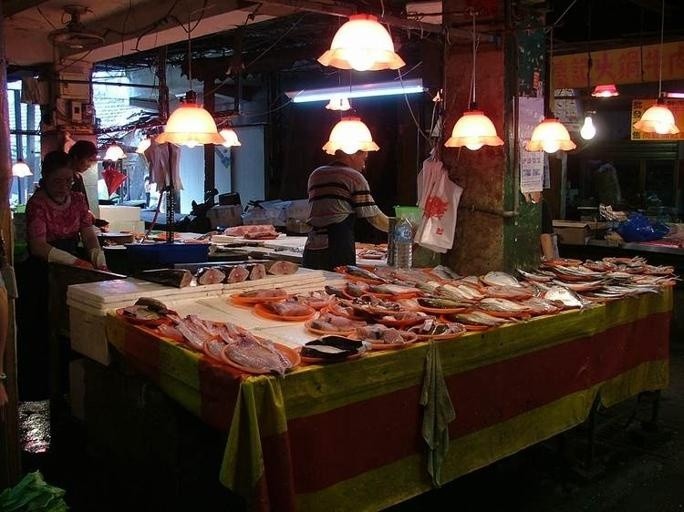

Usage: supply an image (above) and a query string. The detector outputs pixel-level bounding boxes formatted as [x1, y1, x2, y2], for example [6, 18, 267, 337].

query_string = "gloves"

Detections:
[89, 247, 110, 272]
[48, 247, 94, 270]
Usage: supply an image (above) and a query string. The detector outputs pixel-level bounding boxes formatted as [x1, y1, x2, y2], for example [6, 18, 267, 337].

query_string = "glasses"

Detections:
[357, 153, 367, 163]
[48, 176, 75, 187]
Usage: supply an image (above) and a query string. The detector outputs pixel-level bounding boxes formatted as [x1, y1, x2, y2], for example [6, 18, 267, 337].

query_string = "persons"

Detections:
[25, 151, 107, 281]
[68, 140, 99, 225]
[304, 150, 390, 271]
[541, 192, 554, 261]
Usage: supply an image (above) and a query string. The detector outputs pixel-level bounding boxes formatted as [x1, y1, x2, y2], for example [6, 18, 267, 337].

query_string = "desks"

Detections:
[113, 260, 677, 511]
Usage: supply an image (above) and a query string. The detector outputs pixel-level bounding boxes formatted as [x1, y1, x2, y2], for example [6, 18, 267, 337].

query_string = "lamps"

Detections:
[284, 78, 424, 103]
[523, 29, 577, 153]
[580, 50, 596, 140]
[591, 84, 619, 98]
[11, 132, 33, 179]
[154, 11, 225, 149]
[217, 121, 241, 148]
[316, 4, 406, 71]
[324, 70, 351, 111]
[633, 0, 680, 135]
[444, 11, 505, 150]
[102, 141, 126, 163]
[321, 117, 380, 156]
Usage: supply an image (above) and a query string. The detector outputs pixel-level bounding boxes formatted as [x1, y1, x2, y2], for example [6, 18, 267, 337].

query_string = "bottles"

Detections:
[395, 215, 412, 271]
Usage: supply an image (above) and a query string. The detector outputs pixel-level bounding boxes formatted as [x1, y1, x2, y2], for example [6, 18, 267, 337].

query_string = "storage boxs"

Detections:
[551, 220, 590, 246]
[66, 275, 222, 367]
[99, 205, 144, 234]
[206, 204, 242, 229]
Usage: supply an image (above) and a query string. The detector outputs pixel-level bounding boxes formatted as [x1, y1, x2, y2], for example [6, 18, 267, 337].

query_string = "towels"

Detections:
[420, 339, 456, 489]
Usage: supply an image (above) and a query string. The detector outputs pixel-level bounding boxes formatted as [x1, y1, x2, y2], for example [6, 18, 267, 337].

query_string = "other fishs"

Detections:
[416, 298, 475, 308]
[345, 264, 391, 284]
[369, 282, 423, 296]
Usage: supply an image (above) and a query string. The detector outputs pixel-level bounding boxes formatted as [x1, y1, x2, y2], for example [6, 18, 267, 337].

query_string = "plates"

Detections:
[155, 317, 244, 347]
[480, 285, 532, 301]
[371, 314, 436, 327]
[341, 285, 366, 300]
[343, 272, 384, 286]
[362, 285, 418, 298]
[230, 290, 287, 303]
[305, 318, 365, 336]
[293, 344, 366, 362]
[474, 302, 531, 318]
[252, 300, 315, 321]
[405, 321, 465, 340]
[348, 330, 416, 349]
[347, 299, 407, 316]
[440, 312, 497, 331]
[413, 297, 468, 313]
[204, 333, 267, 363]
[115, 307, 180, 325]
[288, 292, 335, 309]
[220, 338, 300, 374]
